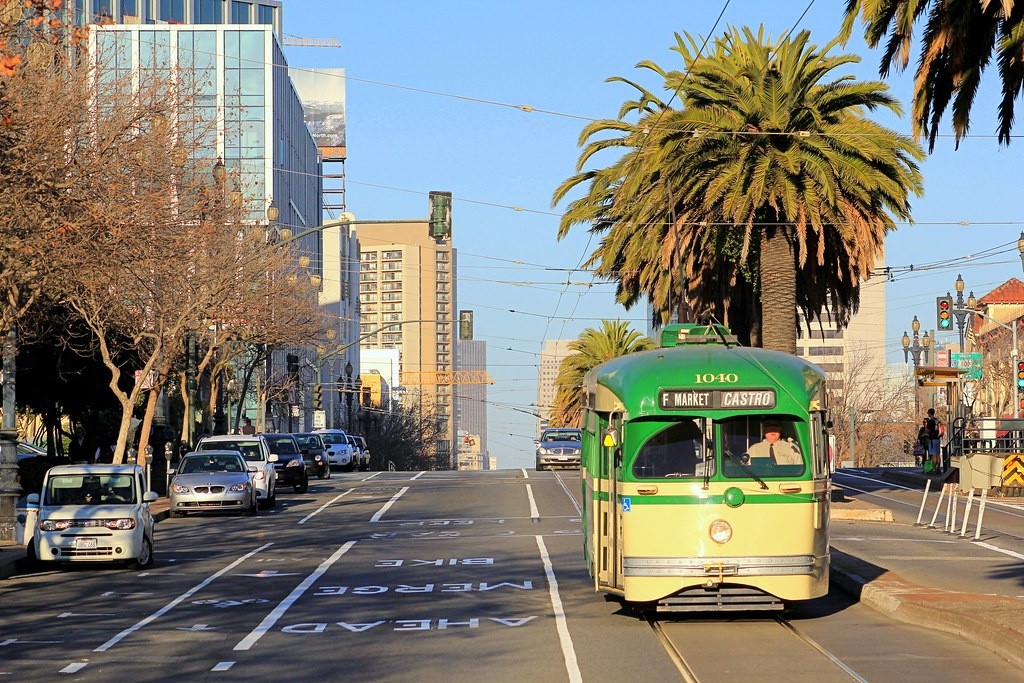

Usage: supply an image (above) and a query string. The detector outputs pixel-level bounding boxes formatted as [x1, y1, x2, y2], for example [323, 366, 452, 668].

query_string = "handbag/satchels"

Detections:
[913, 441, 924, 456]
[923, 458, 935, 475]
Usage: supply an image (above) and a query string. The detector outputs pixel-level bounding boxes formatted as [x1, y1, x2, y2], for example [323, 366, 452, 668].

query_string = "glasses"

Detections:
[927, 411, 929, 414]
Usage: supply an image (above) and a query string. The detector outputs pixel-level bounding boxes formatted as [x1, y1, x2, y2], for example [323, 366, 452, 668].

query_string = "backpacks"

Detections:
[934, 419, 944, 437]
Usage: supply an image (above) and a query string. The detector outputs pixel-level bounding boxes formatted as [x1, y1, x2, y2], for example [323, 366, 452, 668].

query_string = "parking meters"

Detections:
[165, 442, 173, 498]
[127, 448, 136, 464]
[227, 380, 236, 434]
[145, 444, 153, 504]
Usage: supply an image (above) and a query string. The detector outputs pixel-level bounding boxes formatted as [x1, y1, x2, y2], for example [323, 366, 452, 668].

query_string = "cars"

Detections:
[16, 443, 70, 466]
[345, 435, 371, 471]
[27, 464, 159, 569]
[166, 449, 258, 518]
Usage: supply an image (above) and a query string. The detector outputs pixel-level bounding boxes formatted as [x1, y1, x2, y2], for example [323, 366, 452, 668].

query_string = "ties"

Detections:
[768, 444, 776, 466]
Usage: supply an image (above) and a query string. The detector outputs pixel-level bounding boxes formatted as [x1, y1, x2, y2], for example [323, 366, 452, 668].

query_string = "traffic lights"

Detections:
[938, 296, 953, 331]
[286, 353, 299, 382]
[1017, 361, 1024, 393]
[313, 384, 323, 409]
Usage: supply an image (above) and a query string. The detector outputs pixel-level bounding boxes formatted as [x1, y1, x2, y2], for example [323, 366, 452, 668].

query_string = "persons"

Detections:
[667, 420, 713, 474]
[918, 408, 942, 474]
[746, 417, 803, 465]
[570, 435, 577, 440]
[70, 427, 116, 463]
[197, 429, 212, 440]
[134, 416, 158, 461]
[547, 436, 554, 441]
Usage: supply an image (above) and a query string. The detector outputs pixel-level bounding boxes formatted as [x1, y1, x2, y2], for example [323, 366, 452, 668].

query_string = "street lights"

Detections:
[285, 254, 322, 432]
[902, 315, 930, 439]
[357, 395, 380, 448]
[317, 330, 345, 435]
[198, 156, 243, 434]
[256, 202, 292, 432]
[946, 273, 978, 454]
[338, 361, 362, 433]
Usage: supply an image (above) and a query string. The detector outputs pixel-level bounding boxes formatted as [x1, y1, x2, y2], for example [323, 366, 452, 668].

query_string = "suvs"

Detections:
[290, 433, 330, 478]
[312, 428, 353, 471]
[251, 433, 308, 492]
[194, 433, 278, 507]
[534, 428, 583, 471]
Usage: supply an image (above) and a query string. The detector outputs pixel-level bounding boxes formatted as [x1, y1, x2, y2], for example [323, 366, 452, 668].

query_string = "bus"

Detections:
[582, 323, 834, 611]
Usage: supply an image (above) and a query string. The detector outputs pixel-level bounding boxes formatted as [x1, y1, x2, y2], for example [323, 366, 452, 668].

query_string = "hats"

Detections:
[761, 415, 783, 427]
